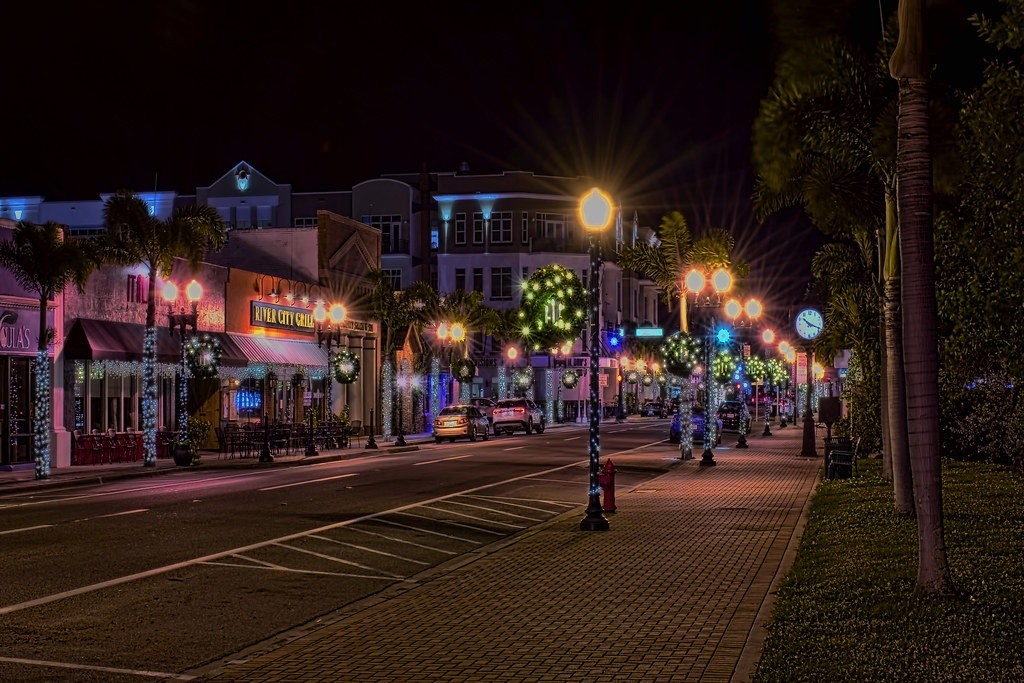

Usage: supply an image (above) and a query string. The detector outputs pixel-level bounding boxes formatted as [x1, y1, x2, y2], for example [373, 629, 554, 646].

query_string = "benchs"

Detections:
[827, 437, 861, 479]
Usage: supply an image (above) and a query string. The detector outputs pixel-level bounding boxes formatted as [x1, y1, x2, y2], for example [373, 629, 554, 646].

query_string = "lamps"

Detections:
[259, 274, 326, 309]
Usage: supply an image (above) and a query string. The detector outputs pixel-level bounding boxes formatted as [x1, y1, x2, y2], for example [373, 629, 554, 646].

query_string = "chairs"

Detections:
[215, 420, 362, 461]
[73, 425, 180, 466]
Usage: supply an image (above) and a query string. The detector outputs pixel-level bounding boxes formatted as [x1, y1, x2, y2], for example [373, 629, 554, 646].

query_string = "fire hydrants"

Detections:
[598, 459, 617, 512]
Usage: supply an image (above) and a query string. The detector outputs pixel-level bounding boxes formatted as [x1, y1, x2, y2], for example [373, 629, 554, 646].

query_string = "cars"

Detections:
[492, 397, 546, 436]
[715, 401, 753, 434]
[668, 407, 721, 445]
[639, 403, 667, 418]
[433, 406, 492, 444]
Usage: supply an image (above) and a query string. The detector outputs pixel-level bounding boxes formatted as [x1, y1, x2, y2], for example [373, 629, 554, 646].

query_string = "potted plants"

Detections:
[174, 416, 213, 466]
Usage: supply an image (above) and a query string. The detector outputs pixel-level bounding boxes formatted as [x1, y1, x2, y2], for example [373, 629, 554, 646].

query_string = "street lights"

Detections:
[161, 279, 202, 446]
[723, 299, 764, 449]
[507, 345, 519, 402]
[761, 326, 798, 438]
[437, 322, 467, 406]
[314, 303, 347, 427]
[683, 268, 734, 469]
[576, 185, 618, 533]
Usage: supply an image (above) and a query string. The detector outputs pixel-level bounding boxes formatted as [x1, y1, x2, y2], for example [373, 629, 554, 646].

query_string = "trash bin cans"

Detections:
[772, 405, 781, 416]
[822, 436, 854, 479]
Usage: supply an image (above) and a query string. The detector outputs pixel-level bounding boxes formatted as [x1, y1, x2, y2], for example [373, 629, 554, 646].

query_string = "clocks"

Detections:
[795, 308, 824, 340]
